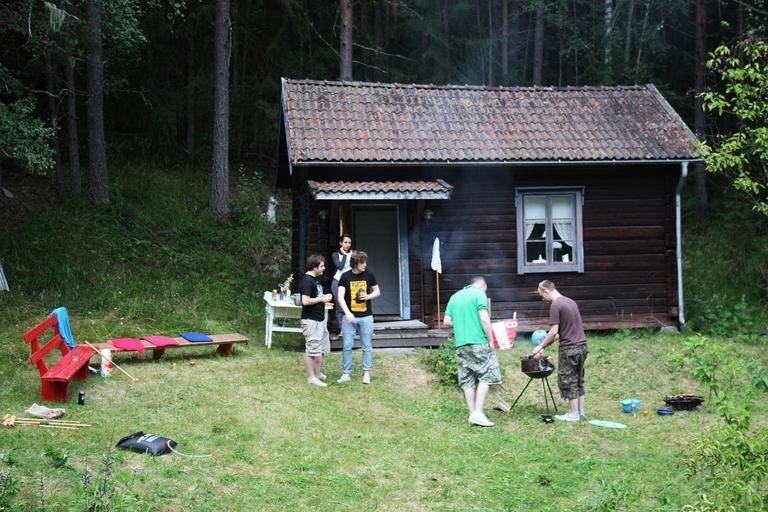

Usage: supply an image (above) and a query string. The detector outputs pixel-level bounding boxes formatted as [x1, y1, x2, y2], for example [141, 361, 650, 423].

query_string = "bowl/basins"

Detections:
[621, 400, 641, 413]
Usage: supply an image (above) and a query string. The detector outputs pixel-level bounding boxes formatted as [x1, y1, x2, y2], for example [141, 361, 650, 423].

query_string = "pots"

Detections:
[292, 293, 301, 306]
[661, 397, 704, 409]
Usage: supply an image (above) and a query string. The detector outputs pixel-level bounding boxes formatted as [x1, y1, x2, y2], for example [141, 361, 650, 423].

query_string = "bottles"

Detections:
[286, 287, 291, 297]
[282, 289, 286, 301]
[78, 390, 84, 405]
[271, 289, 277, 301]
[630, 404, 637, 418]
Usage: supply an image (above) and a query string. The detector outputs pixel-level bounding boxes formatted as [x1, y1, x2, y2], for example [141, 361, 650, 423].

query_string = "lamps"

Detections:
[421, 209, 434, 219]
[318, 209, 328, 220]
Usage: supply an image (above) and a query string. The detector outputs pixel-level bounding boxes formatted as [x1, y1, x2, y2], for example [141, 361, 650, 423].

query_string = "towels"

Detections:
[110, 337, 144, 355]
[178, 332, 212, 342]
[431, 237, 442, 274]
[50, 306, 75, 347]
[142, 334, 179, 348]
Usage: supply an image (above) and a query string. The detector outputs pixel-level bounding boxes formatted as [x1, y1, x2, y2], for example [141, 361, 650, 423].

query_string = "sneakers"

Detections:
[315, 373, 327, 381]
[307, 376, 328, 387]
[337, 373, 351, 384]
[553, 411, 580, 422]
[467, 411, 496, 428]
[362, 371, 371, 384]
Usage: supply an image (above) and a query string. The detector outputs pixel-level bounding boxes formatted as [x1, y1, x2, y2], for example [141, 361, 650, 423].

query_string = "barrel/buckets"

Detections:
[620, 399, 640, 413]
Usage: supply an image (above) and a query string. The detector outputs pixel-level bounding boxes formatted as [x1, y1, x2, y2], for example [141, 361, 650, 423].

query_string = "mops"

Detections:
[429, 237, 442, 330]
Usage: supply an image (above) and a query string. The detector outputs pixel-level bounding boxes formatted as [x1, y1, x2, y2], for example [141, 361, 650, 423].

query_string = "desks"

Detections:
[262, 293, 335, 349]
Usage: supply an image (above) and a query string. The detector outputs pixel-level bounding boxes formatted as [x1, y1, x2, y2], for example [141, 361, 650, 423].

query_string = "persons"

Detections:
[443, 277, 503, 426]
[332, 235, 376, 340]
[299, 253, 334, 387]
[337, 249, 381, 384]
[533, 280, 588, 422]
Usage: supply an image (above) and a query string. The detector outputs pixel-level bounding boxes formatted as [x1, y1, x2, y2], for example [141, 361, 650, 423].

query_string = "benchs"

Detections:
[79, 331, 250, 365]
[20, 307, 98, 403]
